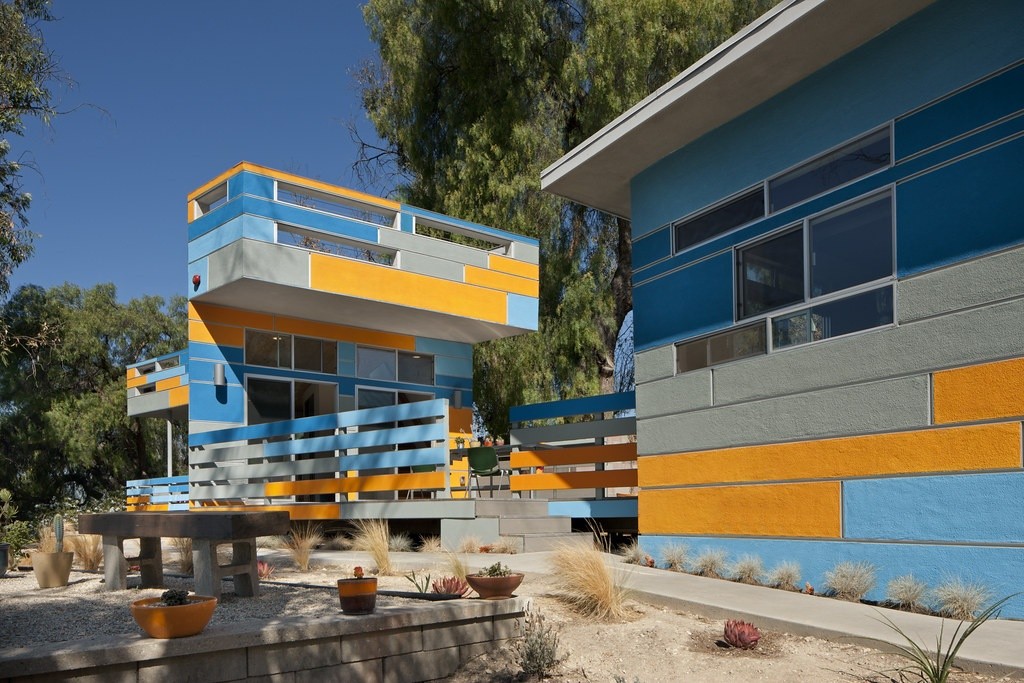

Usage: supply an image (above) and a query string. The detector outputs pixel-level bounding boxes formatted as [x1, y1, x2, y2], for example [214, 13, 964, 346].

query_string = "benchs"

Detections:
[78, 510, 290, 603]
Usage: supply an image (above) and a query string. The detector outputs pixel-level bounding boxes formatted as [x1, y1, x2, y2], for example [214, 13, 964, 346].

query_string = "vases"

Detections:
[471, 442, 481, 448]
[337, 578, 377, 615]
[484, 441, 492, 447]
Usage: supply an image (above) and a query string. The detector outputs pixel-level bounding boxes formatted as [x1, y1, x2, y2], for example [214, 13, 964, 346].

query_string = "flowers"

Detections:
[460, 401, 495, 443]
[354, 567, 365, 579]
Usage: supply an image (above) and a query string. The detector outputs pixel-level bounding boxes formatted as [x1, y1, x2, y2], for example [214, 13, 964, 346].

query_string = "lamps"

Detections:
[214, 363, 226, 386]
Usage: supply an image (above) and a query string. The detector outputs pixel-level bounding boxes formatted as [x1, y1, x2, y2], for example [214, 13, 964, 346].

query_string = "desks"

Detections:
[451, 445, 510, 491]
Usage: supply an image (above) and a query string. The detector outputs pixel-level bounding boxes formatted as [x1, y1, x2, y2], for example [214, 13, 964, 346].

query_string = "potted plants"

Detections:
[455, 437, 464, 448]
[32, 513, 74, 588]
[130, 588, 218, 638]
[465, 561, 525, 600]
[496, 435, 505, 446]
[0, 488, 13, 577]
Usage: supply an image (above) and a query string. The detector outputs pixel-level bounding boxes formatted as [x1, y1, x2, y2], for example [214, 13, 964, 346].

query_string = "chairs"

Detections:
[468, 447, 521, 499]
[406, 465, 446, 499]
